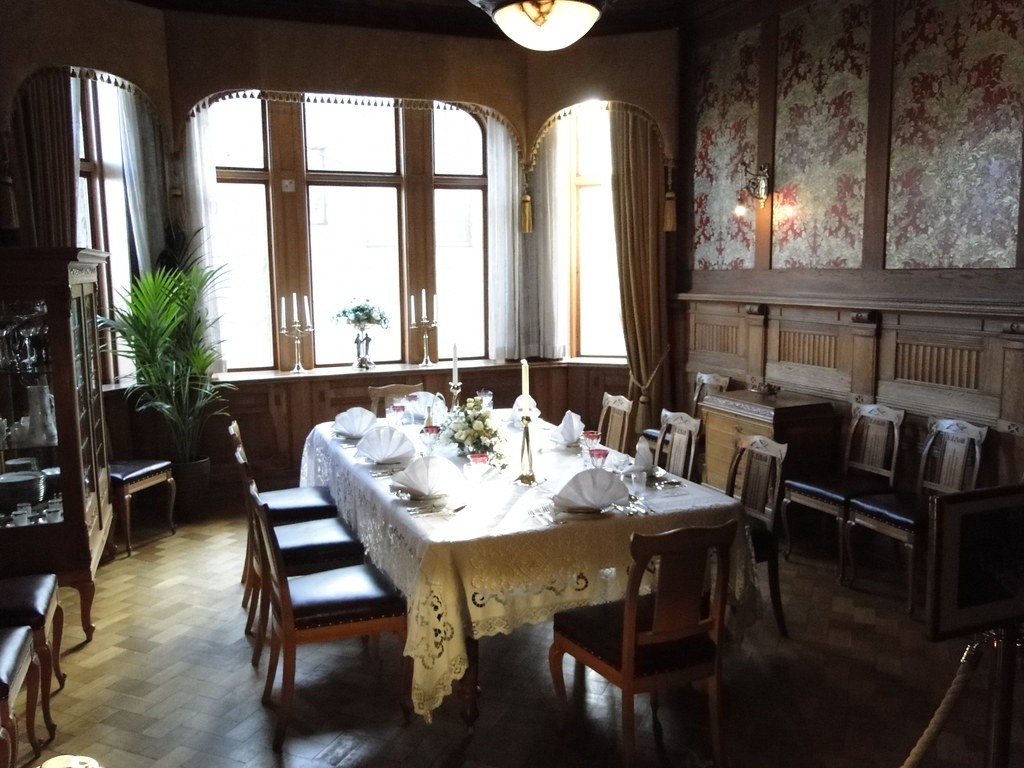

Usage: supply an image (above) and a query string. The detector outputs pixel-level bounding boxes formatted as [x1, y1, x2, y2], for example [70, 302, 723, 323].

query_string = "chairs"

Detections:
[229, 420, 413, 752]
[548, 371, 989, 768]
[104, 418, 177, 557]
[0, 625, 44, 768]
[0, 573, 66, 741]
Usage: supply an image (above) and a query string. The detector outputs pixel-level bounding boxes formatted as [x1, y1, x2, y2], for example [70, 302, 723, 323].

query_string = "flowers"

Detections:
[435, 396, 508, 470]
[333, 299, 390, 329]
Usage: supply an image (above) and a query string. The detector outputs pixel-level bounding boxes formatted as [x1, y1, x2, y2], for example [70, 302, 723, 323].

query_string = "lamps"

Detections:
[734, 160, 770, 217]
[468, 0, 614, 51]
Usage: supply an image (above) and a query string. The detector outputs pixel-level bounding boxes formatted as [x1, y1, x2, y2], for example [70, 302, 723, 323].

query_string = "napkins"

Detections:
[397, 391, 448, 419]
[506, 393, 543, 423]
[352, 425, 416, 464]
[549, 409, 585, 444]
[623, 435, 654, 478]
[548, 469, 630, 513]
[329, 407, 380, 440]
[389, 455, 463, 498]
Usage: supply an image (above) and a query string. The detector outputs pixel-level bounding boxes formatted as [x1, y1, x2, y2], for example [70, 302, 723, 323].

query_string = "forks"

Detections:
[528, 505, 560, 526]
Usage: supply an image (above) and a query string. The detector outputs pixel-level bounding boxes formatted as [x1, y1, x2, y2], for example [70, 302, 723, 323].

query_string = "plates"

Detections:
[11, 513, 39, 518]
[43, 509, 63, 514]
[6, 522, 35, 527]
[5, 458, 39, 472]
[39, 518, 64, 524]
[0, 473, 45, 503]
[41, 467, 62, 499]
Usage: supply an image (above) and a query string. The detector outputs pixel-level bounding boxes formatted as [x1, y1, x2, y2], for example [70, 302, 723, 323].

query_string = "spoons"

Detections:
[414, 505, 467, 518]
[629, 495, 656, 514]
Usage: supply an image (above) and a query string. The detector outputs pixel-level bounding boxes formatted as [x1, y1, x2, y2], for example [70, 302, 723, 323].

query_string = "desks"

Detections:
[299, 409, 761, 736]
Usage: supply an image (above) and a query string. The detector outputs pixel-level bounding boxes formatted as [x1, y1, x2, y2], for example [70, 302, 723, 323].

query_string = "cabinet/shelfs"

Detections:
[0, 246, 119, 642]
[697, 389, 838, 517]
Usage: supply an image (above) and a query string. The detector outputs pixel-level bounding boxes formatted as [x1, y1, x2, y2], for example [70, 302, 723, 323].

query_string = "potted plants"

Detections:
[97, 227, 239, 507]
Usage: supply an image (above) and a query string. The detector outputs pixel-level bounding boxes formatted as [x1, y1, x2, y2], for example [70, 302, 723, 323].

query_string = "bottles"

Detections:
[424, 407, 434, 426]
[26, 385, 57, 445]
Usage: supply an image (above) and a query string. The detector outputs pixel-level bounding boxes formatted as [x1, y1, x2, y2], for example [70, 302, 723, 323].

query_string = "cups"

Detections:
[49, 501, 63, 513]
[632, 472, 646, 497]
[46, 510, 60, 522]
[482, 401, 493, 417]
[17, 504, 31, 515]
[613, 454, 629, 473]
[12, 512, 27, 525]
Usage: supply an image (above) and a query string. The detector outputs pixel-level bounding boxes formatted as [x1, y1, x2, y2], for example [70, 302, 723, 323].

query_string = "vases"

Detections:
[355, 325, 371, 370]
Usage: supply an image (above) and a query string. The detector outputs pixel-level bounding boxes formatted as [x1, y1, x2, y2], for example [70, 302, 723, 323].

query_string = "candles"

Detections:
[520, 359, 530, 415]
[410, 289, 438, 326]
[452, 343, 459, 384]
[281, 292, 311, 330]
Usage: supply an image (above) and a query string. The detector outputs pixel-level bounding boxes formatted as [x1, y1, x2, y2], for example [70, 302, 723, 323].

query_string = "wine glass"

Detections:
[478, 391, 492, 413]
[421, 426, 441, 457]
[463, 455, 487, 496]
[583, 431, 609, 469]
[386, 395, 417, 429]
[0, 297, 51, 370]
